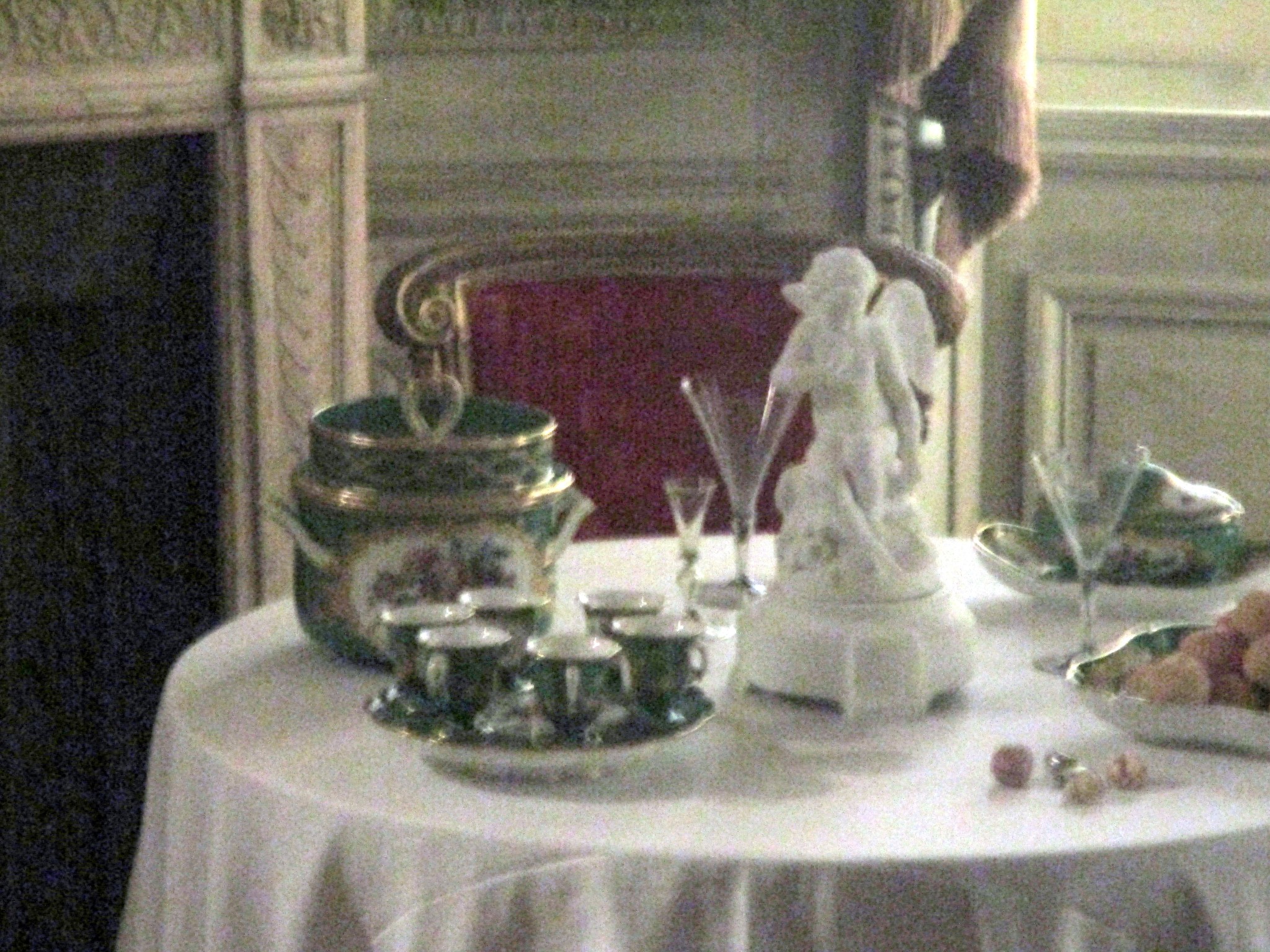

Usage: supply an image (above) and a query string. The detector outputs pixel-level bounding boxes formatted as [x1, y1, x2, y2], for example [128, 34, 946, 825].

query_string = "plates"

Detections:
[1066, 622, 1270, 757]
[364, 686, 718, 781]
[971, 522, 1270, 616]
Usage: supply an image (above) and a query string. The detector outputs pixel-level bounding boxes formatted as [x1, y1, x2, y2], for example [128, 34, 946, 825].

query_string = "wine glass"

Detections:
[681, 375, 802, 612]
[662, 478, 718, 639]
[1030, 446, 1149, 675]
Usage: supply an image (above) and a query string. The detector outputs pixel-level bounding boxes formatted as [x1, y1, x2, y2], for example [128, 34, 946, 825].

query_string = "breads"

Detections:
[1121, 585, 1270, 715]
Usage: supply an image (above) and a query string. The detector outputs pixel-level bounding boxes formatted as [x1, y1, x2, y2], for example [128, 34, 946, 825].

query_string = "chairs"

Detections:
[375, 225, 968, 541]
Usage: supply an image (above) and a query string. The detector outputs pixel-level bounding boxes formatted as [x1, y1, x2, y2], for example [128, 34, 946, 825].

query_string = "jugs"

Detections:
[525, 474, 527, 477]
[258, 375, 596, 673]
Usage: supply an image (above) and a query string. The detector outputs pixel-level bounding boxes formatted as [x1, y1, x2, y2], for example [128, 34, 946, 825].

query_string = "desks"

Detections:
[117, 533, 1270, 952]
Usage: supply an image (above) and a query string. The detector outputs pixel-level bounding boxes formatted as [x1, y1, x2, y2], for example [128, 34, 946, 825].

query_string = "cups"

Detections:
[612, 615, 708, 708]
[456, 587, 550, 672]
[415, 625, 513, 716]
[578, 591, 662, 638]
[525, 635, 623, 732]
[365, 603, 475, 692]
